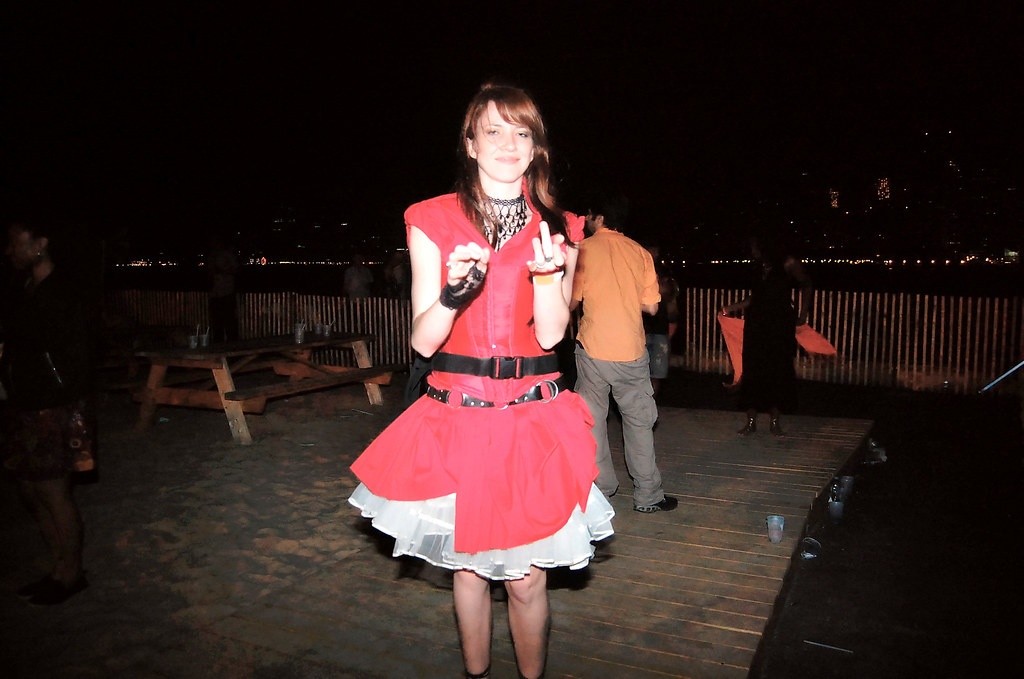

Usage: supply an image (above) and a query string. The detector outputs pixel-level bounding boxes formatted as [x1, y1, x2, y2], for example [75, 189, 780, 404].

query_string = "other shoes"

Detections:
[16, 577, 44, 599]
[28, 572, 85, 607]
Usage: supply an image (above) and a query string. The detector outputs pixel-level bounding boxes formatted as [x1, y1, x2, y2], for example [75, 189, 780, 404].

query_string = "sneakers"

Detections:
[635, 495, 678, 513]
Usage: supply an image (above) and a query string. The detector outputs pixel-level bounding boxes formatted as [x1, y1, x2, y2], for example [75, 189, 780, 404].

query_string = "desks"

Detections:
[130, 332, 380, 451]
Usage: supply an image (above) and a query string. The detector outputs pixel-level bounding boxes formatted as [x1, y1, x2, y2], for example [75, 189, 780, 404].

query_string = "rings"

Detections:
[544, 255, 555, 262]
[537, 264, 547, 269]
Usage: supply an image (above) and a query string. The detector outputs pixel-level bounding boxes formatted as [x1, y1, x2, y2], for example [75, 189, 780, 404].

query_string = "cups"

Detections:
[323, 325, 331, 336]
[767, 516, 784, 544]
[187, 336, 197, 349]
[295, 329, 304, 344]
[199, 335, 209, 347]
[315, 324, 322, 334]
[295, 324, 306, 330]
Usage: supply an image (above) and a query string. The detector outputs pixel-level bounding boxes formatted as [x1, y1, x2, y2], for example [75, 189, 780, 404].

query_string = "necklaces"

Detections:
[482, 193, 527, 253]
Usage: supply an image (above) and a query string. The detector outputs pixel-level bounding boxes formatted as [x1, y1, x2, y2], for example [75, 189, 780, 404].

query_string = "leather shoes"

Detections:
[737, 417, 755, 434]
[769, 417, 784, 438]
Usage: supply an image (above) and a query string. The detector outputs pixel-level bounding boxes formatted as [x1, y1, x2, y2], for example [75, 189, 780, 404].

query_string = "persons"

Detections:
[347, 80, 615, 679]
[721, 230, 812, 438]
[0, 219, 88, 609]
[569, 199, 679, 513]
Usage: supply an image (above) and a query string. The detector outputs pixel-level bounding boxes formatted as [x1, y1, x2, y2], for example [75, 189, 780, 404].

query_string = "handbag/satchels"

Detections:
[67, 408, 95, 473]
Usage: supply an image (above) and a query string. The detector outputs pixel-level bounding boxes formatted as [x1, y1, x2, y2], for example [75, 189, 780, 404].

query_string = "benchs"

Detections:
[113, 359, 272, 393]
[223, 363, 406, 401]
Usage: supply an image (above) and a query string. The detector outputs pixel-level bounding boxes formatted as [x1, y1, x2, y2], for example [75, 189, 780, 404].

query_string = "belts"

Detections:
[424, 376, 565, 407]
